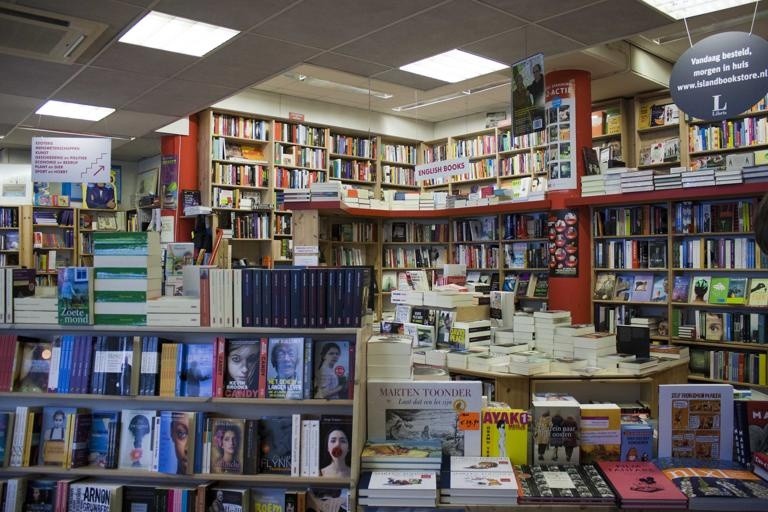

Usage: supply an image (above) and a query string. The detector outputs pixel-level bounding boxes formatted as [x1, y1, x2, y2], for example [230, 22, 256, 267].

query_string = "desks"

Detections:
[372, 307, 692, 426]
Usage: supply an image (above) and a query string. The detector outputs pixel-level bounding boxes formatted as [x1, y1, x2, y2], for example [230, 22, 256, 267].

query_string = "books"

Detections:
[210, 110, 429, 326]
[419, 100, 767, 511]
[2, 333, 441, 512]
[1, 206, 138, 324]
[56, 229, 219, 326]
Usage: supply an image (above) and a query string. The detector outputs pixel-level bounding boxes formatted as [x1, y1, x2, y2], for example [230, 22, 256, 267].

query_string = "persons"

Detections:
[511, 74, 531, 109]
[527, 63, 544, 103]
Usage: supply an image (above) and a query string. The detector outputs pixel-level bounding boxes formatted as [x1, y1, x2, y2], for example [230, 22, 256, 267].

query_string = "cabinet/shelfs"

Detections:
[79, 207, 126, 265]
[633, 83, 768, 173]
[128, 198, 161, 237]
[29, 205, 78, 272]
[589, 199, 669, 344]
[0, 202, 28, 271]
[669, 188, 768, 386]
[591, 94, 630, 173]
[212, 108, 552, 314]
[1, 319, 374, 512]
